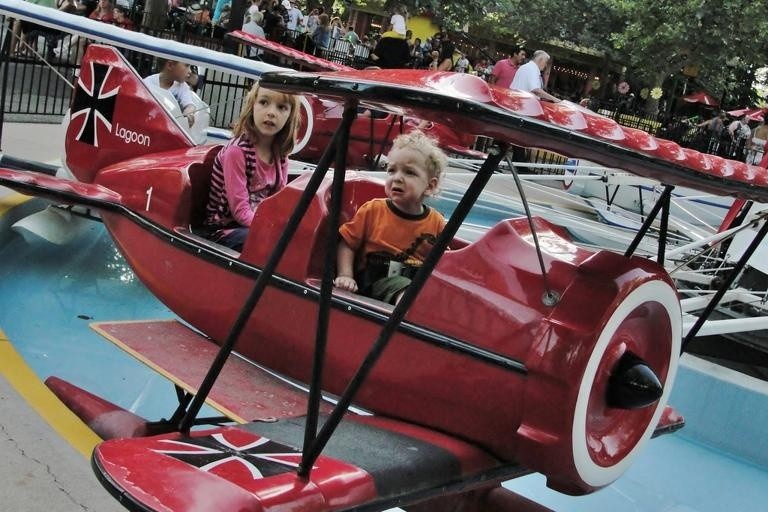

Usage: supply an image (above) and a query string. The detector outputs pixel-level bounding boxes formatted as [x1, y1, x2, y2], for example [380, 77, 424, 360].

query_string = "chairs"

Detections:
[318, 38, 336, 60]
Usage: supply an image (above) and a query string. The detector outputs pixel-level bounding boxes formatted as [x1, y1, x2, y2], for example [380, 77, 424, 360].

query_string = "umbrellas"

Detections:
[681, 89, 720, 107]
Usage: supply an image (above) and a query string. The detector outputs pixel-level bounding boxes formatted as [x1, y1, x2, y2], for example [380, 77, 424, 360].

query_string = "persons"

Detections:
[331, 126, 452, 306]
[509, 50, 562, 175]
[141, 58, 199, 128]
[5, 0, 496, 84]
[201, 76, 302, 252]
[696, 110, 768, 167]
[488, 45, 526, 90]
